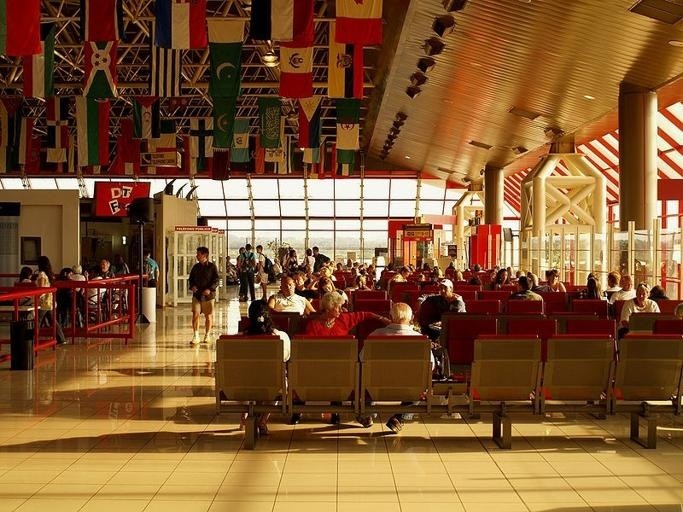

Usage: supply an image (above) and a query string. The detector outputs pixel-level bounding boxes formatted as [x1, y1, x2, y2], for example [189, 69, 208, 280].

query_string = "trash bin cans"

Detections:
[142, 287, 157, 324]
[9, 320, 35, 370]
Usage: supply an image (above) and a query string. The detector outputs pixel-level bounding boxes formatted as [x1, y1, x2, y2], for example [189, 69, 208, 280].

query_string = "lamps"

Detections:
[261, 41, 281, 66]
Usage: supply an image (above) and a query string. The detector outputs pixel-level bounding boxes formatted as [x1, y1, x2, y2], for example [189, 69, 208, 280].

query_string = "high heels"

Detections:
[240, 416, 247, 431]
[255, 418, 270, 435]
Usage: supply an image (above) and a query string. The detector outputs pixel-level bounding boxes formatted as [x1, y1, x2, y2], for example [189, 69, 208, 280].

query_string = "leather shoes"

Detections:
[330, 412, 341, 424]
[292, 411, 303, 424]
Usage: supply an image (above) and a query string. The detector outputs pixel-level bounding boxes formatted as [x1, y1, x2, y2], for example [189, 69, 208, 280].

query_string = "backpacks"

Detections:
[242, 253, 254, 273]
[259, 252, 272, 274]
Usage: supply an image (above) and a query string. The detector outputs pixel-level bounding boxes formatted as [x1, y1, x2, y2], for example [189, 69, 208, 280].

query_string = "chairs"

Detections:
[211, 271, 683, 452]
[40, 281, 130, 331]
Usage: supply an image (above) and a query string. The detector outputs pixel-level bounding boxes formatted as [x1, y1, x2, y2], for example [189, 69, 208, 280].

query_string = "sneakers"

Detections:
[203, 335, 210, 342]
[190, 336, 200, 345]
[386, 415, 403, 433]
[239, 296, 268, 302]
[56, 339, 67, 348]
[354, 412, 373, 427]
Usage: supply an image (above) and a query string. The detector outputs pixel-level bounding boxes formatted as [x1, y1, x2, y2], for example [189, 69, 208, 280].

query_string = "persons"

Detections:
[188, 247, 219, 346]
[143, 251, 159, 287]
[20, 255, 131, 344]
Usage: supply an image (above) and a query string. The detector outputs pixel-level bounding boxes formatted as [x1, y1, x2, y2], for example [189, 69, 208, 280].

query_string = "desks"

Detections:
[0, 305, 41, 314]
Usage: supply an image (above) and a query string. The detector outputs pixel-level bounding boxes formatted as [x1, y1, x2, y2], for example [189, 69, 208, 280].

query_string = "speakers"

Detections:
[129, 197, 155, 225]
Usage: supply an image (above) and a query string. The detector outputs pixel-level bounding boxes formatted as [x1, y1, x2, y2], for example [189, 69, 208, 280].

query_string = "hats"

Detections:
[439, 279, 454, 289]
